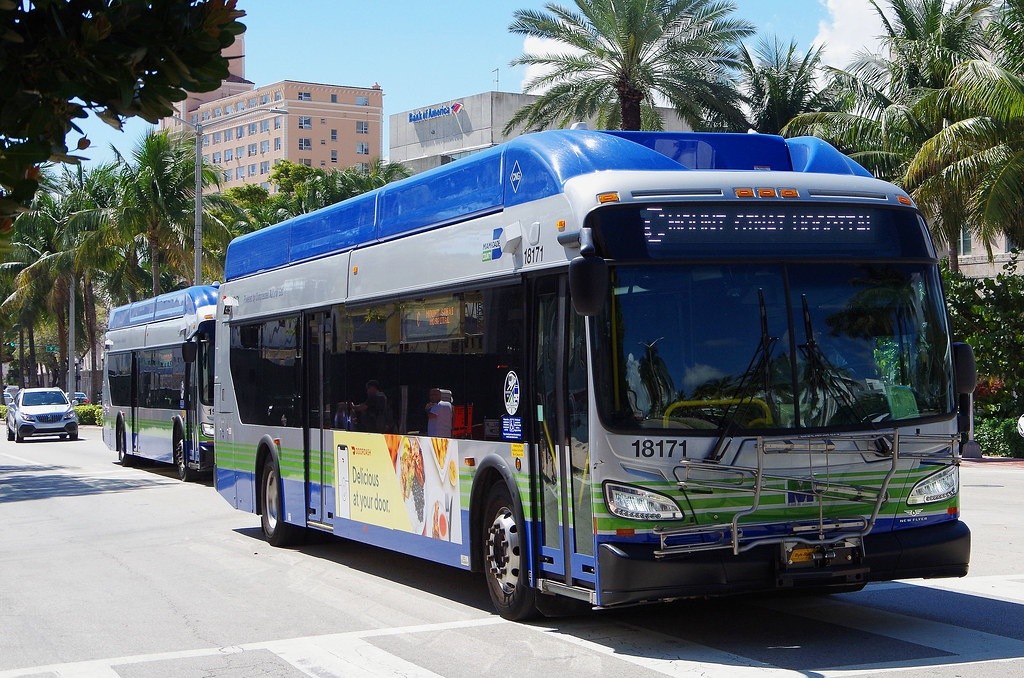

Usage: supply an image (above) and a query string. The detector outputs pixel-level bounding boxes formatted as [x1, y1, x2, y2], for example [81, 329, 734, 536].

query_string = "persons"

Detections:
[425, 388, 452, 438]
[350, 380, 395, 434]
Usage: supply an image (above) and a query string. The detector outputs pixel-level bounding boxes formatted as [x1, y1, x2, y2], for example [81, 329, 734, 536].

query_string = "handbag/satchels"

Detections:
[362, 401, 380, 430]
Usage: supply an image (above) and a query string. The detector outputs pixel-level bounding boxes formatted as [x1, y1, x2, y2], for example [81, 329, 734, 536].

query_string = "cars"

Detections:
[3, 392, 13, 405]
[65, 391, 92, 406]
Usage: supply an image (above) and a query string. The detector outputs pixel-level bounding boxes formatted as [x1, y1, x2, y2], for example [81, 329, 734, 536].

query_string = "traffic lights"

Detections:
[3, 342, 16, 348]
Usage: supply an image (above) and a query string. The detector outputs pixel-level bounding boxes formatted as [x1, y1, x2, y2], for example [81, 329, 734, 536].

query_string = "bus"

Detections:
[215, 129, 981, 622]
[101, 279, 221, 483]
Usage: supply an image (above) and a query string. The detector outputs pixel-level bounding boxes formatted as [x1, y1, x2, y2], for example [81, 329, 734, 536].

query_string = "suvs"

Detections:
[5, 387, 79, 443]
